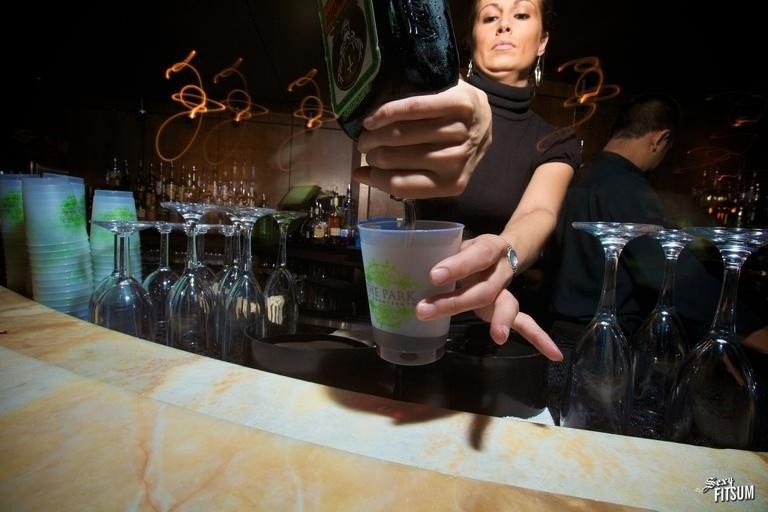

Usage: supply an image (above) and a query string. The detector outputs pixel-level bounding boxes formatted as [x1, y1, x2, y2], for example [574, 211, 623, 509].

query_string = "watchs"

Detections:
[503, 242, 520, 279]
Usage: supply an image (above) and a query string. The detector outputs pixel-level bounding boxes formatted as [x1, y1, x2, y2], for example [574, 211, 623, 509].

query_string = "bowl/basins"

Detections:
[243, 313, 378, 389]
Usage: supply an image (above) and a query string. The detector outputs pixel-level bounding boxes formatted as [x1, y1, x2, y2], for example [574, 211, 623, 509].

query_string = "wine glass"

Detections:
[562, 221, 768, 453]
[90, 198, 306, 368]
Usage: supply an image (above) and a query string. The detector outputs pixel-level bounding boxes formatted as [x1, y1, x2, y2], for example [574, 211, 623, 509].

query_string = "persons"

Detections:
[353, 0, 590, 368]
[543, 88, 729, 344]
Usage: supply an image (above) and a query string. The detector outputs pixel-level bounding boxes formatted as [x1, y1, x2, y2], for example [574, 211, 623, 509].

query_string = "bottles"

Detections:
[694, 168, 763, 227]
[93, 154, 256, 220]
[308, 0, 458, 200]
[301, 179, 354, 238]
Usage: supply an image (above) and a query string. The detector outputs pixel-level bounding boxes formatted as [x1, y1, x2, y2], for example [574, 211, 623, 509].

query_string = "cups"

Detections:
[356, 220, 464, 366]
[3, 171, 142, 330]
